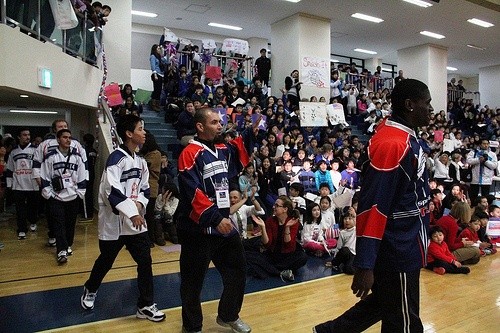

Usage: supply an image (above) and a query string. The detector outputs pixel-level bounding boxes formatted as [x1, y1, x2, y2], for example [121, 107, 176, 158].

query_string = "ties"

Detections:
[235, 211, 243, 236]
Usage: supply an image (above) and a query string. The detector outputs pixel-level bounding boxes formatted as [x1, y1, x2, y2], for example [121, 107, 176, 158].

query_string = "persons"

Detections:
[41, 129, 86, 262]
[0, 133, 97, 222]
[111, 83, 180, 247]
[0, 0, 111, 67]
[313, 79, 434, 333]
[330, 61, 500, 274]
[173, 107, 252, 333]
[31, 119, 89, 246]
[6, 129, 41, 239]
[149, 32, 357, 280]
[81, 113, 166, 321]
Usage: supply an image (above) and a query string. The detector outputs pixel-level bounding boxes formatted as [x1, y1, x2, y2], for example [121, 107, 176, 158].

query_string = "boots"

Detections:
[150, 98, 161, 112]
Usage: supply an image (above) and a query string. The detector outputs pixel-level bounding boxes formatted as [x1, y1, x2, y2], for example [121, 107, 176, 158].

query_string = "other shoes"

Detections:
[462, 267, 470, 274]
[434, 267, 445, 274]
[280, 270, 293, 283]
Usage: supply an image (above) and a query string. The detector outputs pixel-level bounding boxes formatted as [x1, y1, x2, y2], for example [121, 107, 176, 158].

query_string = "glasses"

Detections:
[276, 204, 283, 208]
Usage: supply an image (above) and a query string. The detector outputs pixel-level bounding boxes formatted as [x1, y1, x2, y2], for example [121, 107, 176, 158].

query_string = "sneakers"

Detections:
[17, 232, 26, 240]
[216, 315, 251, 333]
[136, 303, 166, 321]
[80, 285, 98, 310]
[48, 237, 56, 246]
[57, 250, 68, 263]
[29, 223, 37, 232]
[67, 247, 73, 255]
